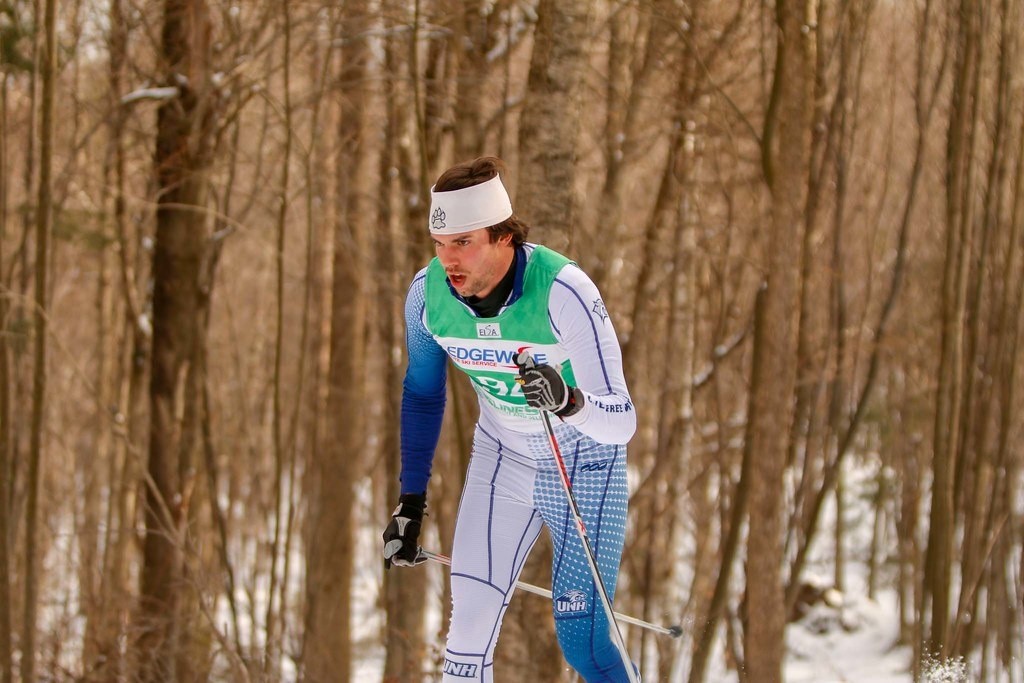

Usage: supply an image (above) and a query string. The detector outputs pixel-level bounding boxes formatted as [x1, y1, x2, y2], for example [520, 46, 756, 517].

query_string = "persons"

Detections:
[383, 157, 640, 683]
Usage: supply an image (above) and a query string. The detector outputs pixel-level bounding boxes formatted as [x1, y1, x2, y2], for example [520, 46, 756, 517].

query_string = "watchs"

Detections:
[565, 388, 584, 416]
